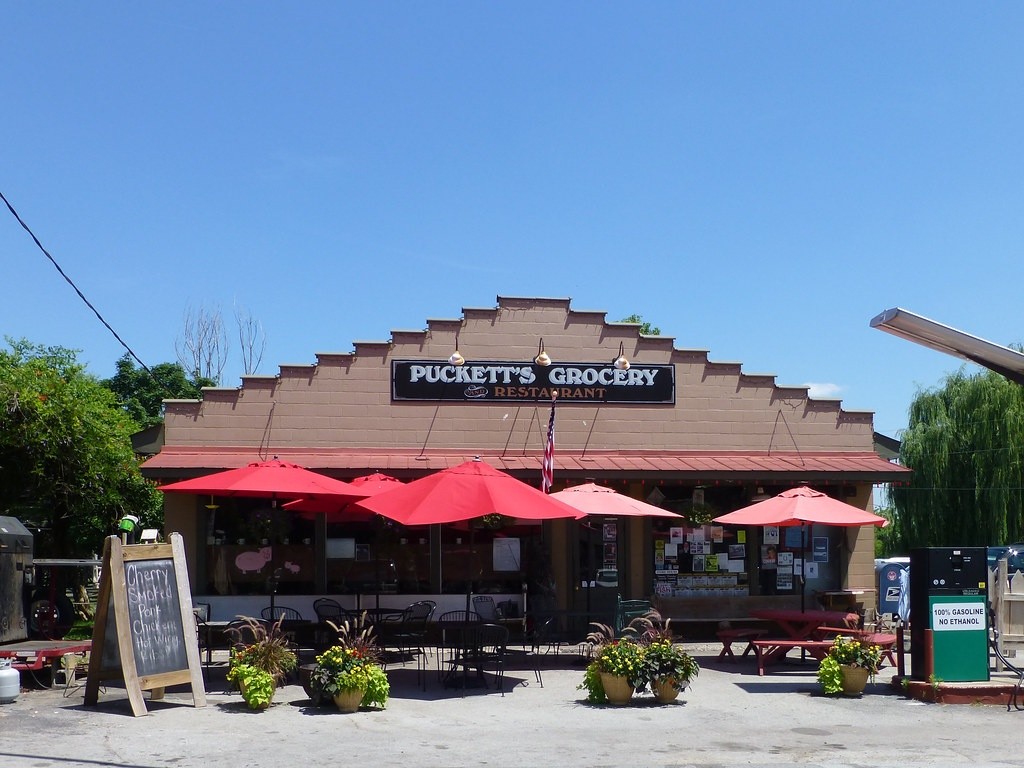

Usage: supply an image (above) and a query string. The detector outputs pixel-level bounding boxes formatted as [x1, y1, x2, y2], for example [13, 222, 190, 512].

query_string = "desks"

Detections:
[345, 608, 414, 626]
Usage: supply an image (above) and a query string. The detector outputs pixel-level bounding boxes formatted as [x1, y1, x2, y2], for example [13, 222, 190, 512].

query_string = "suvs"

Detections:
[986, 544, 1024, 580]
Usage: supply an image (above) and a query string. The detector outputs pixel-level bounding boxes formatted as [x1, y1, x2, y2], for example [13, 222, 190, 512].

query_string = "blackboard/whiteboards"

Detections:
[85, 532, 207, 687]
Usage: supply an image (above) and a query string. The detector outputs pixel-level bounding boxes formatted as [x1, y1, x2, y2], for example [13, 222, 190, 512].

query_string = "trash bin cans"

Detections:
[819, 592, 857, 628]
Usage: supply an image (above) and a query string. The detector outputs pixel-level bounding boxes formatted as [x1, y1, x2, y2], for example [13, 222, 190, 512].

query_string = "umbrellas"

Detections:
[547, 481, 684, 517]
[710, 485, 891, 666]
[353, 454, 589, 638]
[279, 473, 407, 615]
[155, 453, 373, 623]
[540, 400, 556, 494]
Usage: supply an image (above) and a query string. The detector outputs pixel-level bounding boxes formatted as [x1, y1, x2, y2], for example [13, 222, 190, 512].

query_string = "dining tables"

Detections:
[749, 608, 859, 665]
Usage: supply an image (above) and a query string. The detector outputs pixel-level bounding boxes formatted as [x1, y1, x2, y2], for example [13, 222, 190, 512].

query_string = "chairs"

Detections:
[191, 598, 557, 697]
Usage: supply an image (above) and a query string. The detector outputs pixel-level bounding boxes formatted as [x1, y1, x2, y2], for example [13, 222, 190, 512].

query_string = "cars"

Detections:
[596, 568, 619, 588]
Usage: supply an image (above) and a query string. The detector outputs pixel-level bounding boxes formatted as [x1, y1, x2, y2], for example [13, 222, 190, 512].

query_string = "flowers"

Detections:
[818, 635, 881, 694]
[224, 608, 301, 709]
[576, 636, 701, 706]
[309, 610, 389, 708]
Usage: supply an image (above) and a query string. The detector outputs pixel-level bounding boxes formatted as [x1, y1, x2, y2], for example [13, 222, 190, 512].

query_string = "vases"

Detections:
[240, 679, 275, 711]
[653, 669, 683, 704]
[333, 685, 365, 713]
[599, 670, 634, 706]
[836, 664, 869, 697]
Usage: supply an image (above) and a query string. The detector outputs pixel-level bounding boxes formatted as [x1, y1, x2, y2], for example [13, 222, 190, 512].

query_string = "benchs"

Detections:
[752, 640, 836, 677]
[716, 628, 768, 666]
[818, 627, 898, 668]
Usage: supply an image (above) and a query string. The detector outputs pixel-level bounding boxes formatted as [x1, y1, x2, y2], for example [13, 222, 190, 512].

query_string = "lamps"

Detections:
[448, 336, 464, 366]
[612, 341, 630, 370]
[532, 337, 551, 367]
